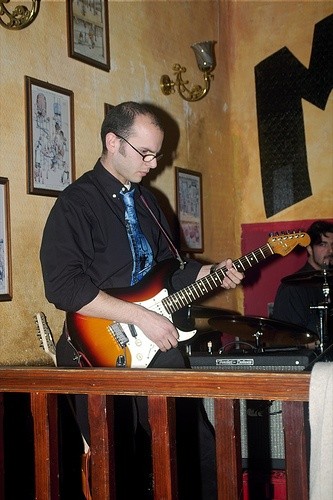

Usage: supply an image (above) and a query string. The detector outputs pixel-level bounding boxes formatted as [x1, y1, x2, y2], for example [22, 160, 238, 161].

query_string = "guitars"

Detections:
[65, 229, 311, 369]
[33, 311, 93, 500]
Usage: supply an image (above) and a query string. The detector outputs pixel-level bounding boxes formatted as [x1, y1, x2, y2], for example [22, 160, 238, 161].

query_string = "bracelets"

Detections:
[210, 263, 219, 274]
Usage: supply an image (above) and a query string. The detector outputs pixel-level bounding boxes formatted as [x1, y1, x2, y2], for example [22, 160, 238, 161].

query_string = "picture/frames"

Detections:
[175, 166, 205, 254]
[0, 177, 13, 302]
[24, 75, 75, 197]
[65, 0, 111, 73]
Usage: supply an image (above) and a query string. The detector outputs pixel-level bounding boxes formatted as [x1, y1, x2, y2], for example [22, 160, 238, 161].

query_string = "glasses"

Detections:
[116, 134, 164, 162]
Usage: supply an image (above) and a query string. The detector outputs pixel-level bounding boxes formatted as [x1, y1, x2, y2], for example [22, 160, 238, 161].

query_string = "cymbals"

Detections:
[281, 269, 333, 288]
[186, 304, 242, 319]
[208, 316, 319, 345]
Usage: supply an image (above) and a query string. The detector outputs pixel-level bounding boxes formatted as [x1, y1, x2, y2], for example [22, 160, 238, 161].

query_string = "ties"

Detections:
[121, 188, 153, 286]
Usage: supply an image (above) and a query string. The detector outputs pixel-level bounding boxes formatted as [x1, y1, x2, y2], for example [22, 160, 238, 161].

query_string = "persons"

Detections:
[40, 101, 244, 500]
[273, 220, 333, 371]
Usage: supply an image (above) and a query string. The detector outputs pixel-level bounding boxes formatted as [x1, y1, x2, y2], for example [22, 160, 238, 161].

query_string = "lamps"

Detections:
[160, 40, 218, 103]
